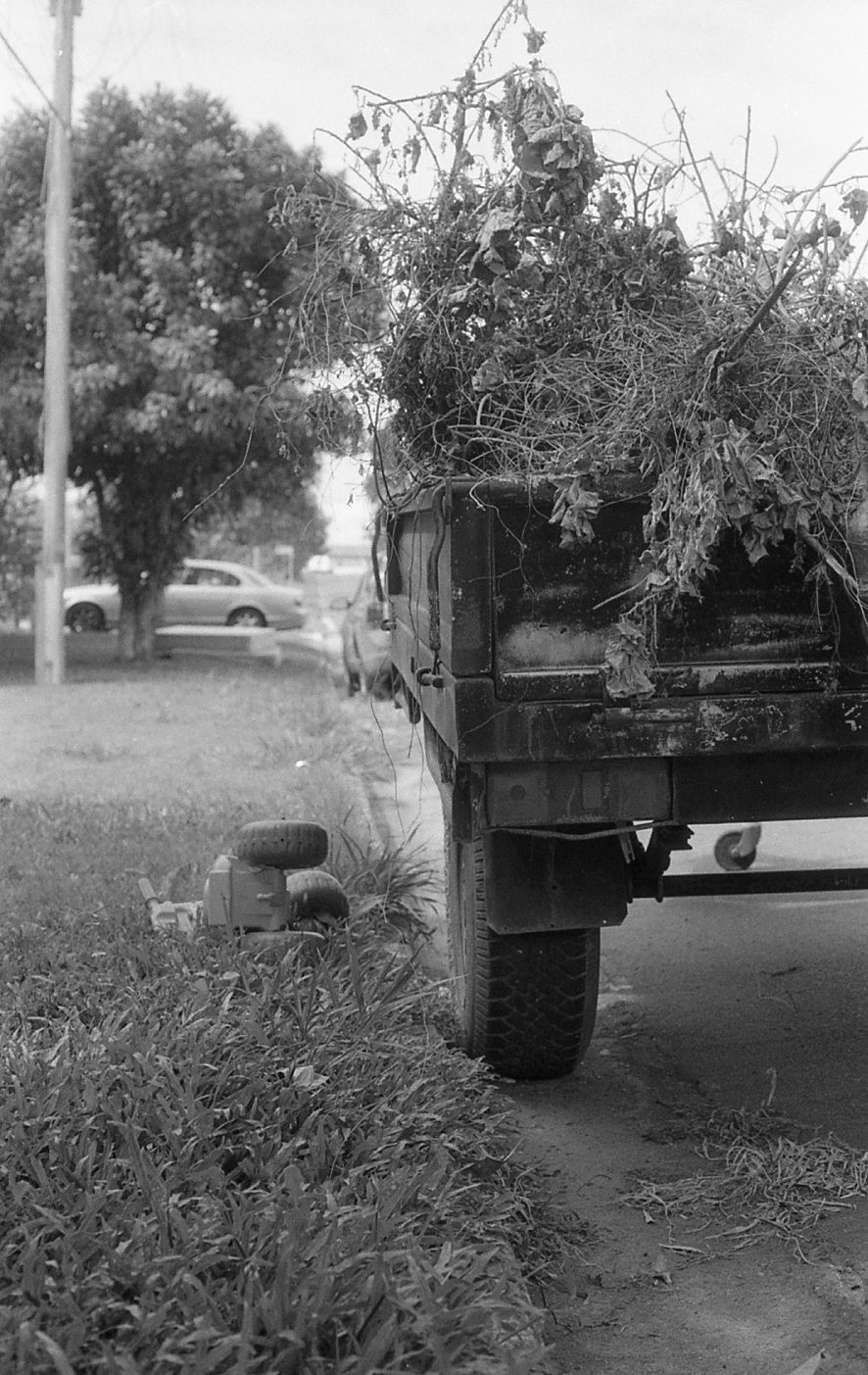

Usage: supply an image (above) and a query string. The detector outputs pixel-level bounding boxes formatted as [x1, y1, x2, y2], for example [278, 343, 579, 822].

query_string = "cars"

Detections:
[61, 558, 305, 633]
[332, 571, 409, 699]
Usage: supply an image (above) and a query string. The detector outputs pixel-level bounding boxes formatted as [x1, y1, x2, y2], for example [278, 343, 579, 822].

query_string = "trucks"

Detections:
[374, 477, 868, 1081]
